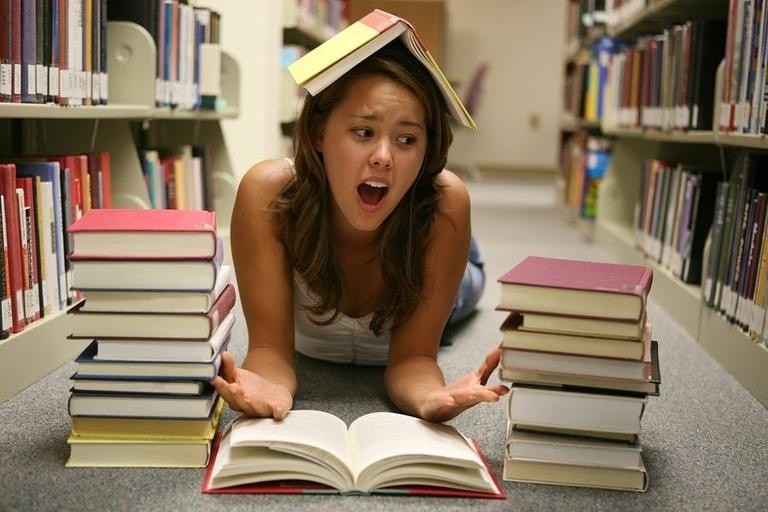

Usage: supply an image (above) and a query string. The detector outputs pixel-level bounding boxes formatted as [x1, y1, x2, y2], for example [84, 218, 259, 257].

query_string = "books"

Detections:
[204, 409, 507, 499]
[1, 142, 215, 340]
[495, 255, 662, 492]
[281, 0, 351, 62]
[288, 10, 483, 132]
[66, 208, 238, 468]
[564, 0, 767, 347]
[110, 1, 220, 112]
[0, 0, 108, 107]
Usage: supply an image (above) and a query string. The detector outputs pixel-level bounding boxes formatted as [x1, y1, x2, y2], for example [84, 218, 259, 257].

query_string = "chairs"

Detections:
[447, 64, 489, 181]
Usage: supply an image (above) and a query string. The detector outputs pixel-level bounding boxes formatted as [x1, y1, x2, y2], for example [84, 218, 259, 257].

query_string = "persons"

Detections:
[211, 58, 509, 422]
